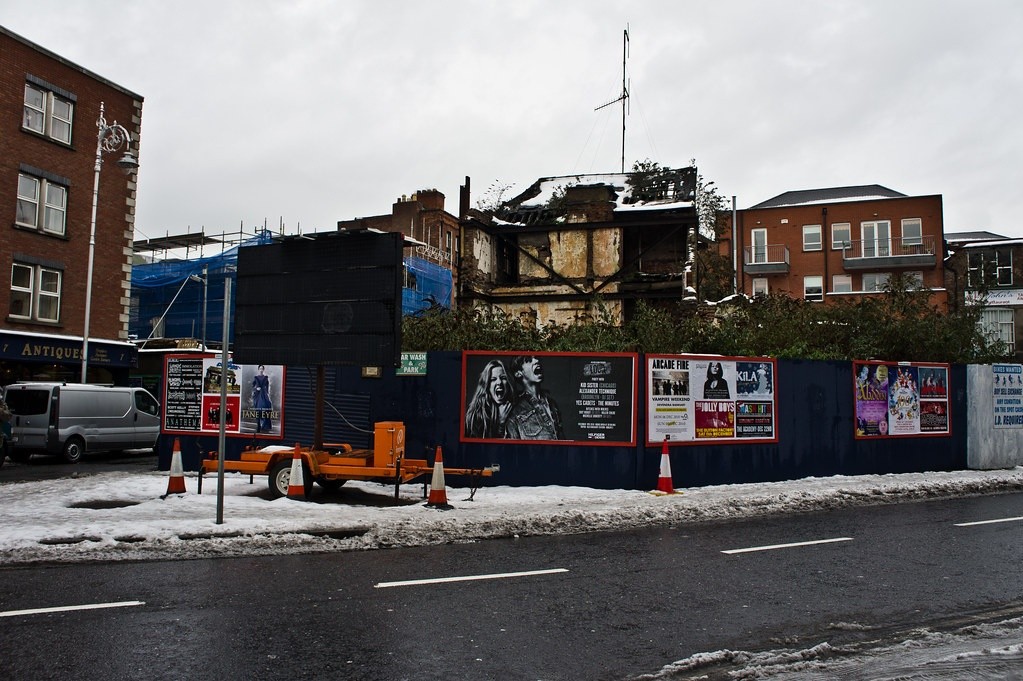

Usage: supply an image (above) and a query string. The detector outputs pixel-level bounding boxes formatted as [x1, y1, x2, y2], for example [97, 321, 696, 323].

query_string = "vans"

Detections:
[3, 383, 161, 463]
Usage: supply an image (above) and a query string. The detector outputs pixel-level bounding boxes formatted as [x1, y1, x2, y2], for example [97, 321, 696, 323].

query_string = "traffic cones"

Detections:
[160, 438, 188, 496]
[424, 447, 453, 510]
[652, 439, 681, 496]
[284, 444, 308, 501]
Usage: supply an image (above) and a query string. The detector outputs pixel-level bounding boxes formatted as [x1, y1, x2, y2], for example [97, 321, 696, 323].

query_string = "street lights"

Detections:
[81, 124, 142, 383]
